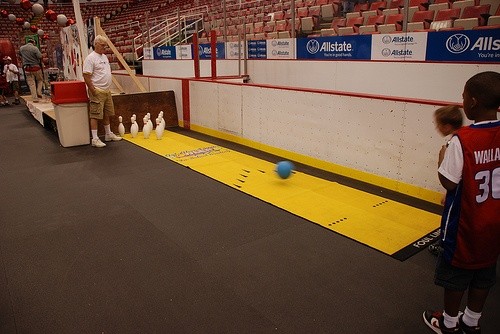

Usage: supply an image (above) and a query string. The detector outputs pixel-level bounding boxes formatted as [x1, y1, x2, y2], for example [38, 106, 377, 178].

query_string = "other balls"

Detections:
[277, 161, 292, 179]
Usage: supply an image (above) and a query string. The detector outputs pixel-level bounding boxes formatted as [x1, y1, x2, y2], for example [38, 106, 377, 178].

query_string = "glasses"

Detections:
[98, 43, 107, 47]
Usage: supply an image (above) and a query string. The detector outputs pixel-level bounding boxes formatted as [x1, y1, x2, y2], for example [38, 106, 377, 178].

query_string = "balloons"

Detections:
[0, 0, 75, 39]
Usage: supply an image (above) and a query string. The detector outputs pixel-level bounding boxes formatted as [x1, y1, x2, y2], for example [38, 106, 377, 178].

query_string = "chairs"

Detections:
[0, 0, 500, 69]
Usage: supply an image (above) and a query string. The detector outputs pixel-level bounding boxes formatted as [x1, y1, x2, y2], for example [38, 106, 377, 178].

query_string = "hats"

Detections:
[27, 37, 35, 45]
[2, 56, 12, 61]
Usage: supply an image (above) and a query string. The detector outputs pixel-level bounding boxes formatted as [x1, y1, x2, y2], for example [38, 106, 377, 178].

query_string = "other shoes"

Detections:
[428, 244, 444, 257]
[33, 100, 39, 103]
[37, 91, 42, 98]
[0, 103, 9, 107]
[12, 99, 20, 105]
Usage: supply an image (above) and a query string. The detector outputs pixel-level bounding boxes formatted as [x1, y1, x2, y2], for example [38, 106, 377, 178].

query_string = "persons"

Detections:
[20, 39, 45, 103]
[0, 56, 20, 106]
[83, 35, 123, 148]
[427, 105, 464, 256]
[423, 70, 500, 334]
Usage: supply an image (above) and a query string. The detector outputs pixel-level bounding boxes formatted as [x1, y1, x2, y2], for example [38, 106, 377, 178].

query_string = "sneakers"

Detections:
[422, 310, 461, 334]
[459, 311, 482, 334]
[92, 138, 107, 148]
[105, 132, 123, 141]
